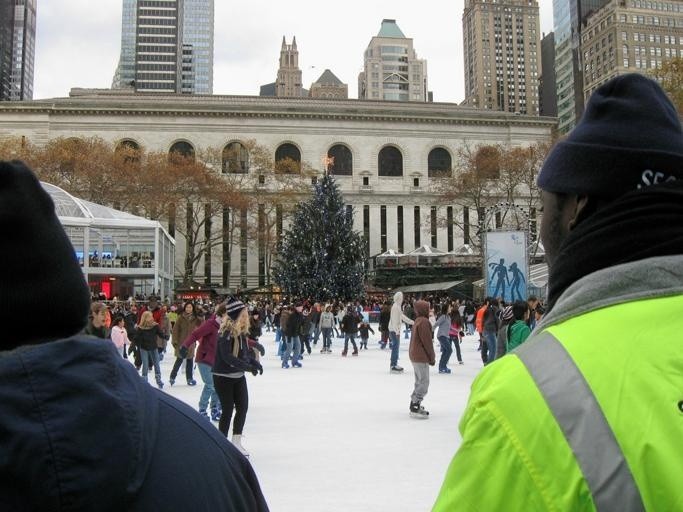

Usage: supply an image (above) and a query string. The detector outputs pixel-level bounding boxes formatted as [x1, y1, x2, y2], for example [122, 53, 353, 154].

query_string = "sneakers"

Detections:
[199, 409, 209, 418]
[232, 435, 250, 456]
[380, 341, 395, 349]
[156, 379, 164, 386]
[439, 366, 452, 373]
[459, 360, 464, 364]
[186, 378, 196, 384]
[410, 401, 429, 415]
[211, 409, 223, 420]
[320, 346, 333, 354]
[392, 366, 404, 371]
[281, 359, 303, 370]
[342, 349, 359, 356]
[170, 377, 175, 384]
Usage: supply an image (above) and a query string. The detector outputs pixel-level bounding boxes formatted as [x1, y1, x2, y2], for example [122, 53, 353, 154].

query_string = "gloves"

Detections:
[245, 360, 263, 376]
[178, 346, 189, 359]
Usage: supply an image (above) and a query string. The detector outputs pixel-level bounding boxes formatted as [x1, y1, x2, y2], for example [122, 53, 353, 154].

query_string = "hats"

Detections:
[91, 302, 111, 311]
[0, 159, 92, 351]
[536, 72, 682, 196]
[503, 304, 515, 320]
[251, 308, 260, 316]
[295, 301, 303, 308]
[226, 298, 246, 320]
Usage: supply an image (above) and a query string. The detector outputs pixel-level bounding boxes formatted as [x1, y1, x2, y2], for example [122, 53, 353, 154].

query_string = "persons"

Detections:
[180, 305, 264, 423]
[0, 160, 273, 511]
[267, 290, 415, 372]
[210, 297, 263, 460]
[87, 250, 148, 267]
[429, 73, 683, 511]
[422, 293, 544, 372]
[408, 299, 436, 418]
[82, 290, 272, 389]
